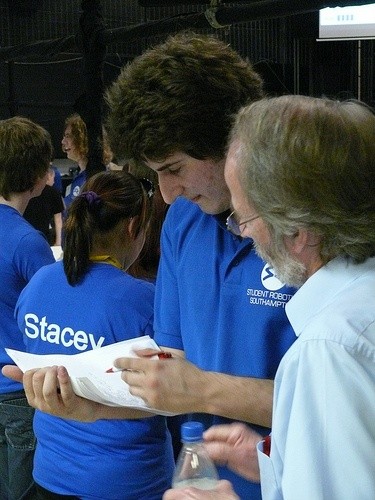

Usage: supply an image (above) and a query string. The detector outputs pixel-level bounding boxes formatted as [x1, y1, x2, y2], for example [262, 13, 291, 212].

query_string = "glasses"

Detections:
[225, 211, 281, 235]
[134, 178, 155, 240]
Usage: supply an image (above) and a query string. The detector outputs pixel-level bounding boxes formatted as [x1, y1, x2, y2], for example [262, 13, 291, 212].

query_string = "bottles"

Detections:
[171, 420, 220, 487]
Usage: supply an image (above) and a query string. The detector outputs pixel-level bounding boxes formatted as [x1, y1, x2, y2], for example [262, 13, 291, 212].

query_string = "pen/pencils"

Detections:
[106, 350, 172, 373]
[262, 437, 271, 457]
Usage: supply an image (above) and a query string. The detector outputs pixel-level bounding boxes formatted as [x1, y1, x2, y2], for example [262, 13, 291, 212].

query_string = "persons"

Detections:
[2, 30, 302, 500]
[162, 94, 375, 500]
[0, 116, 55, 500]
[21, 113, 171, 285]
[14, 171, 157, 500]
